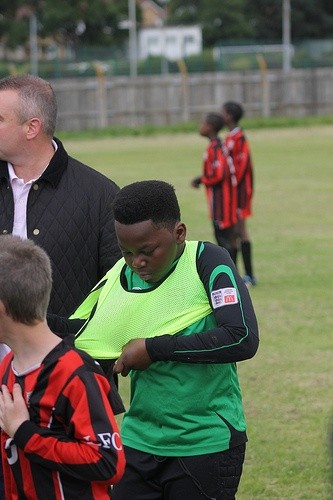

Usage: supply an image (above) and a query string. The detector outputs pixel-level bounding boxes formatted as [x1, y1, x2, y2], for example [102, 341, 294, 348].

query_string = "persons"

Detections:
[221, 101, 256, 288]
[192, 114, 238, 270]
[0, 73, 124, 416]
[57, 180, 259, 499]
[0, 234, 126, 500]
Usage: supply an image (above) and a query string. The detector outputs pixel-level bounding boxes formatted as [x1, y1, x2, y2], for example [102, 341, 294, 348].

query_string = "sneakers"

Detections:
[243, 275, 255, 286]
